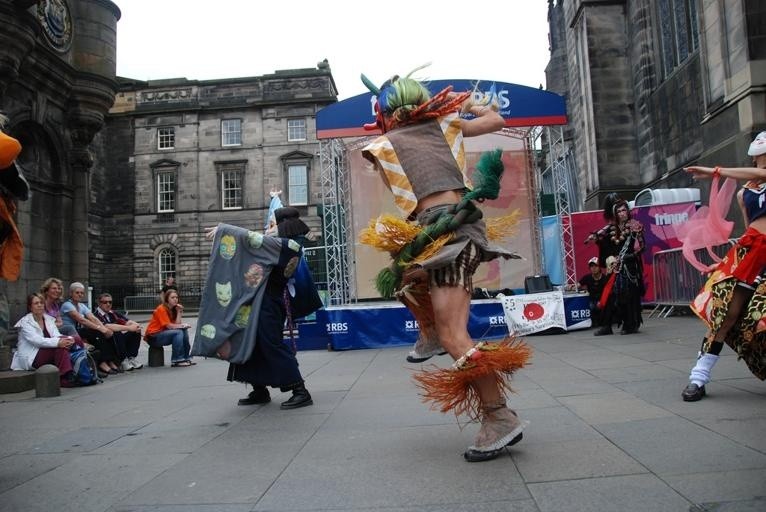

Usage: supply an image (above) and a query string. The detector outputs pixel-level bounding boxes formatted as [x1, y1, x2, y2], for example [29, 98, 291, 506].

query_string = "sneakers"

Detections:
[129, 358, 143, 369]
[407, 322, 450, 362]
[463, 397, 525, 462]
[119, 358, 134, 371]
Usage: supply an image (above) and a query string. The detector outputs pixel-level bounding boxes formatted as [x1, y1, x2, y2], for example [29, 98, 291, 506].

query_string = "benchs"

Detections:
[1, 370, 36, 395]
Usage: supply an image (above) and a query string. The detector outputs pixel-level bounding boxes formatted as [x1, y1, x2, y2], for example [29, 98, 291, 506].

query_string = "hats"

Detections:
[588, 257, 599, 266]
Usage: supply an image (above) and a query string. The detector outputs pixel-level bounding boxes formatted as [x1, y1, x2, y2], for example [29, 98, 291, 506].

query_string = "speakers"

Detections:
[524, 275, 553, 294]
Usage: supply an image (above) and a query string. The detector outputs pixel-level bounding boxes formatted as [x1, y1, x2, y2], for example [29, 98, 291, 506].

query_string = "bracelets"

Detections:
[476, 104, 488, 116]
[712, 166, 720, 179]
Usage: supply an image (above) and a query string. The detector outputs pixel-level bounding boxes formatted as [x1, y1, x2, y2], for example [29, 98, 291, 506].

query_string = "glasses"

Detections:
[99, 300, 112, 304]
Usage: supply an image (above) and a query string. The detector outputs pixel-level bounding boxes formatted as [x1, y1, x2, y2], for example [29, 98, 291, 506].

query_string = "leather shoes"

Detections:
[281, 390, 313, 408]
[238, 389, 271, 403]
[98, 366, 117, 374]
[682, 383, 706, 401]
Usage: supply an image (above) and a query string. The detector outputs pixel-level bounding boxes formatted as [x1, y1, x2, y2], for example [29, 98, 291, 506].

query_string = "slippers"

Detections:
[172, 361, 190, 367]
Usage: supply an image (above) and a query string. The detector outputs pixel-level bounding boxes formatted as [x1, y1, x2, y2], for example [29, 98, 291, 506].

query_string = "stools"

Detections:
[148, 346, 164, 367]
[35, 364, 60, 398]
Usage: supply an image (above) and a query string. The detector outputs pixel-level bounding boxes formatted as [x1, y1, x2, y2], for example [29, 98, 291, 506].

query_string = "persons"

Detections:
[681, 131, 766, 401]
[1, 112, 30, 372]
[360, 60, 529, 463]
[143, 289, 197, 367]
[195, 188, 313, 409]
[593, 192, 646, 336]
[572, 256, 607, 330]
[10, 277, 143, 387]
[160, 277, 178, 304]
